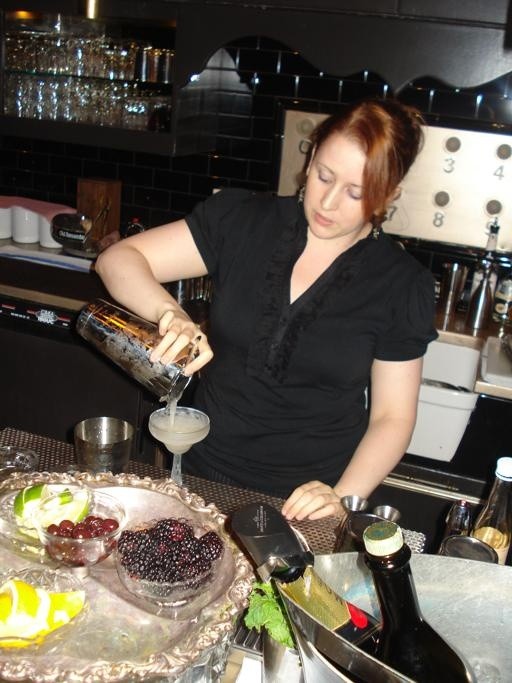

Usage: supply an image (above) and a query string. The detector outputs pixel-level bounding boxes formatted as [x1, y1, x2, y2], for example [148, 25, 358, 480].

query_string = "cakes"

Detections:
[488, 257, 511, 326]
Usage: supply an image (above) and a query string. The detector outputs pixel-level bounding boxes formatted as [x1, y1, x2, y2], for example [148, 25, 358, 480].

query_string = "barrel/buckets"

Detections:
[285, 550, 512, 683]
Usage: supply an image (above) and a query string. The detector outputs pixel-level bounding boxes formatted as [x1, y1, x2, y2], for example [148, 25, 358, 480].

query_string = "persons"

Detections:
[94, 98, 438, 522]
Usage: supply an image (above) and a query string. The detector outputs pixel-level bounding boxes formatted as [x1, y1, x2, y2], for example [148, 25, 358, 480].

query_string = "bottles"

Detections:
[464, 218, 512, 330]
[443, 456, 512, 565]
[123, 217, 144, 236]
[234, 503, 478, 683]
[336, 496, 401, 537]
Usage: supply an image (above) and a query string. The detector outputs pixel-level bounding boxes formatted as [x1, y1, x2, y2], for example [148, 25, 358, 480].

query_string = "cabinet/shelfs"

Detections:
[123, 217, 144, 236]
[464, 218, 512, 330]
[336, 496, 401, 537]
[443, 456, 512, 565]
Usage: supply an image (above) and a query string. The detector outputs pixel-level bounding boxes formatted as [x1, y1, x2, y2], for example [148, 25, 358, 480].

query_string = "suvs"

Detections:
[285, 550, 512, 683]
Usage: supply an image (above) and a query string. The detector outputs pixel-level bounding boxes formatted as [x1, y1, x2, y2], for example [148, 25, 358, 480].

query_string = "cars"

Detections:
[0, 26, 176, 132]
[440, 261, 468, 306]
[0, 196, 81, 247]
[436, 535, 499, 565]
[78, 298, 198, 401]
[75, 416, 135, 478]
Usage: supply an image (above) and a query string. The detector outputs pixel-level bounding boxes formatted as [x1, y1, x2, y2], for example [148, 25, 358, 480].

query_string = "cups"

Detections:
[0, 196, 81, 247]
[436, 535, 499, 565]
[0, 26, 176, 132]
[440, 261, 468, 306]
[75, 416, 135, 478]
[78, 298, 198, 401]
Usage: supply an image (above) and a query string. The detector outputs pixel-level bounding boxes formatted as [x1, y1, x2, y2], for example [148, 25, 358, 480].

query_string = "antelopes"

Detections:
[47, 516, 118, 568]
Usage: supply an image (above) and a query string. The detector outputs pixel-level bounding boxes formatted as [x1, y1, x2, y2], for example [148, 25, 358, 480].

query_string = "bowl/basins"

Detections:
[0, 474, 92, 556]
[111, 512, 224, 601]
[37, 491, 128, 565]
[0, 557, 90, 650]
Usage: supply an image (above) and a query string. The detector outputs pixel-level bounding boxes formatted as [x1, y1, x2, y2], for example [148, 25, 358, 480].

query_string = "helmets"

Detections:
[0, 446, 38, 474]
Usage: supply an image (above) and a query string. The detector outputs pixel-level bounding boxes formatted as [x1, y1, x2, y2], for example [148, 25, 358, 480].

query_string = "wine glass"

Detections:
[149, 406, 212, 487]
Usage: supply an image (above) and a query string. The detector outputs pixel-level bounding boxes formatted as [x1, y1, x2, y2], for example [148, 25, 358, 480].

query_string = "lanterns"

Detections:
[13, 483, 89, 538]
[0, 578, 87, 650]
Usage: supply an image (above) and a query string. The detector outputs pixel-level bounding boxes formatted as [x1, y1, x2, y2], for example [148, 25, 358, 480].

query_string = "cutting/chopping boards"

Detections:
[74, 177, 120, 254]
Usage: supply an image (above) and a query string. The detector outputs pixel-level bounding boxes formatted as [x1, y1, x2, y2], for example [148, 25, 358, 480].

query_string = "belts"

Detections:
[149, 406, 212, 487]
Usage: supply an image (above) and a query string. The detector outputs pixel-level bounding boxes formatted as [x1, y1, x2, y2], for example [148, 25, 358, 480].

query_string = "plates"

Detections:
[1, 1, 218, 156]
[0, 446, 38, 474]
